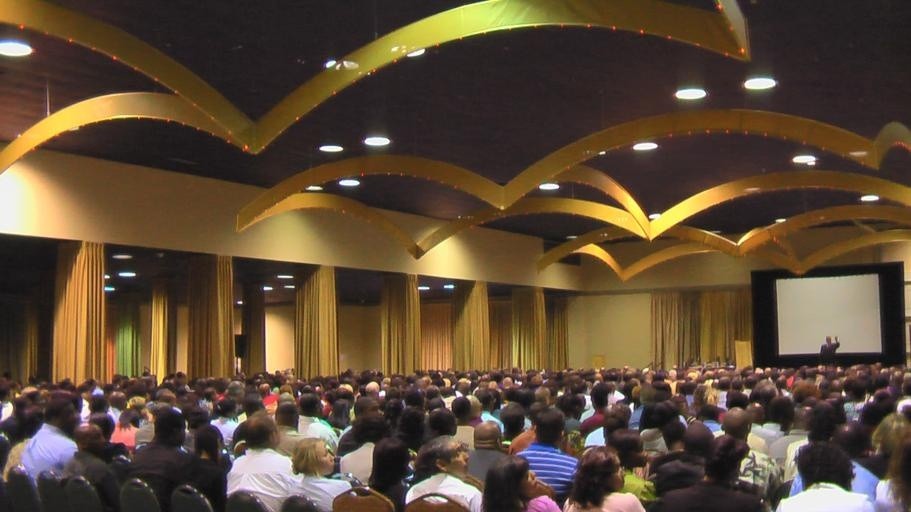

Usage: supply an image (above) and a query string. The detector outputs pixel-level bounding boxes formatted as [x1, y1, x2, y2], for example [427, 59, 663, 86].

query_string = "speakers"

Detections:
[234, 334, 247, 358]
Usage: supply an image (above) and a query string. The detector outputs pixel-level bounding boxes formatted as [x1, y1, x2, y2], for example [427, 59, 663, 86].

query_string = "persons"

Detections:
[820, 336, 840, 364]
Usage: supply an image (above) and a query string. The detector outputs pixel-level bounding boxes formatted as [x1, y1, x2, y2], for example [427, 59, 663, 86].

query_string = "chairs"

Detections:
[1, 438, 554, 512]
